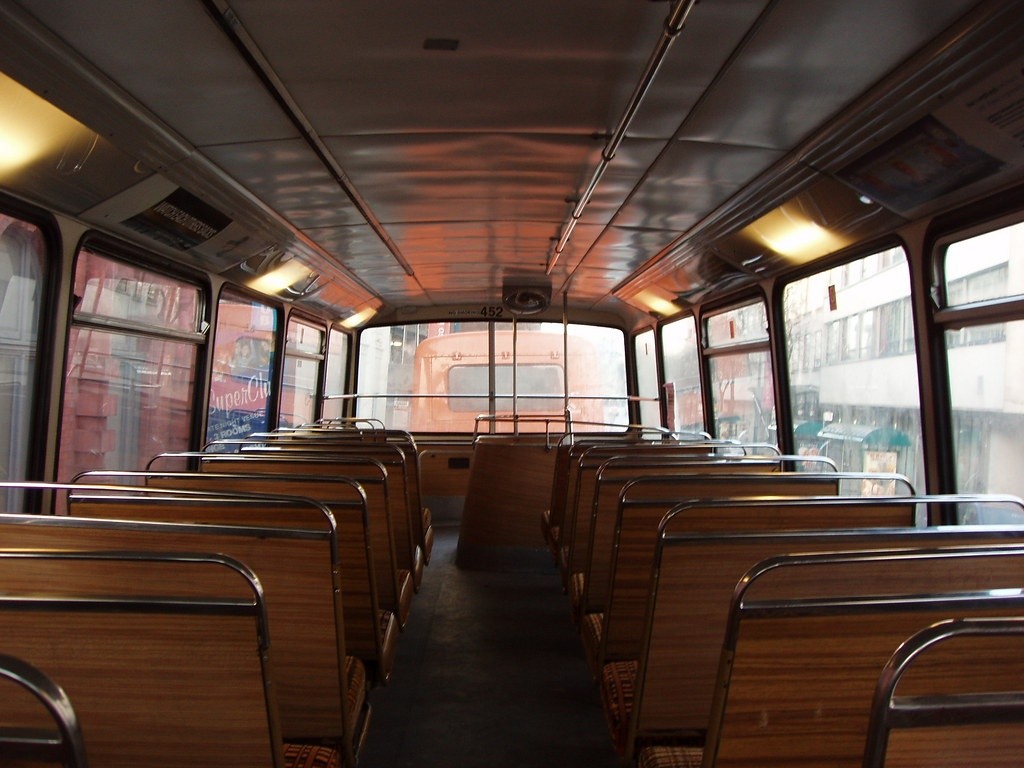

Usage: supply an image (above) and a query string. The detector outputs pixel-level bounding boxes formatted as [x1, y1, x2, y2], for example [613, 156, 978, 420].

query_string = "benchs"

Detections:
[0, 427, 433, 768]
[542, 429, 1024, 768]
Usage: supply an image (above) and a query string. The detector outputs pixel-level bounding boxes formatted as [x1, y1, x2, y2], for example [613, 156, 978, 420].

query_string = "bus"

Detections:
[407, 328, 605, 431]
